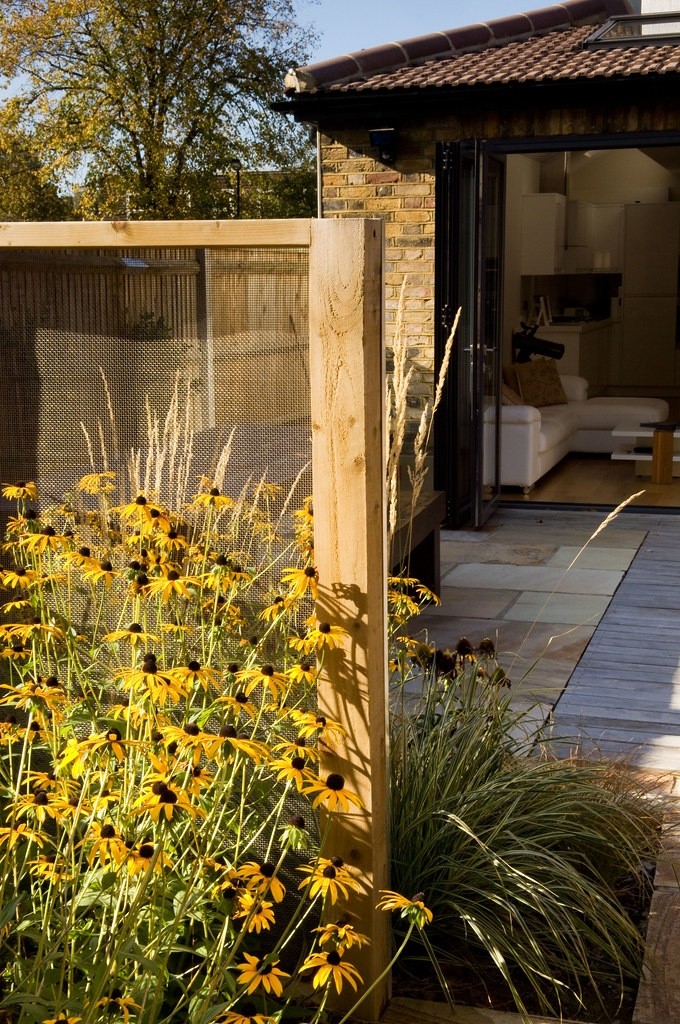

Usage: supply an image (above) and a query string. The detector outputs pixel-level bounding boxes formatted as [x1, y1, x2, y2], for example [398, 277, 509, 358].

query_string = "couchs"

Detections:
[482, 375, 671, 494]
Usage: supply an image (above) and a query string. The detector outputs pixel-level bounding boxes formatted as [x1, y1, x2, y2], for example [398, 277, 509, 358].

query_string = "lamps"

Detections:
[369, 126, 409, 165]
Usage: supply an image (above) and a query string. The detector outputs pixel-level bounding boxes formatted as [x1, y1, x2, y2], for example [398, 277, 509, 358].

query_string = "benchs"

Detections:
[249, 489, 447, 604]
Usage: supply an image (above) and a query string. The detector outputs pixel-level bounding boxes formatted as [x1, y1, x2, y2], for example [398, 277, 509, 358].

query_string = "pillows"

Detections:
[515, 356, 562, 407]
[516, 358, 568, 406]
[504, 363, 521, 396]
[502, 383, 525, 406]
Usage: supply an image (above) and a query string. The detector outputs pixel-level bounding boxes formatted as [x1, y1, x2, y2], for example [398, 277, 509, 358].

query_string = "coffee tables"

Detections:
[611, 422, 680, 485]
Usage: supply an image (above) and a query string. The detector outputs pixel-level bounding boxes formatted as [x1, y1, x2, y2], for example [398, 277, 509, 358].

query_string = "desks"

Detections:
[513, 320, 613, 399]
[47, 426, 312, 555]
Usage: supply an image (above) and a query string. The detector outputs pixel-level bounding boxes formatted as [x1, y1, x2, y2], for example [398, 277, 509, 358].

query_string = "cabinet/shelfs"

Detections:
[524, 192, 680, 400]
